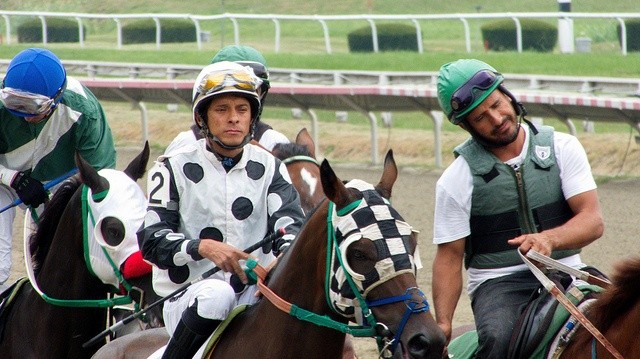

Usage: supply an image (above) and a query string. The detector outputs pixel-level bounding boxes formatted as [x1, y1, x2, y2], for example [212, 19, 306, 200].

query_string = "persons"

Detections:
[0, 46, 117, 285]
[138, 61, 307, 359]
[431, 58, 604, 358]
[164, 44, 291, 159]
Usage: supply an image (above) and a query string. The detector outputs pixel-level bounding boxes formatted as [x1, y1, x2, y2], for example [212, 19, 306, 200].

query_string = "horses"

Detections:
[0, 139, 166, 359]
[438, 254, 640, 359]
[254, 120, 327, 217]
[92, 148, 447, 359]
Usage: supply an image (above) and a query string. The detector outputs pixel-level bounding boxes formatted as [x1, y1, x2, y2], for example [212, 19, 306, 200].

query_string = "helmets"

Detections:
[437, 59, 504, 125]
[210, 46, 270, 101]
[192, 62, 262, 128]
[1, 49, 66, 118]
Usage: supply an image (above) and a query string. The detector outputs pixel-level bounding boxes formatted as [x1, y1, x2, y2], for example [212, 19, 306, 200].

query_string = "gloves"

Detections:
[12, 171, 50, 210]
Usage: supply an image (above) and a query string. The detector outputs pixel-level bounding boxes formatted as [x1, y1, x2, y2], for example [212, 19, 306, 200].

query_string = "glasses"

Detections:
[446, 69, 503, 121]
[232, 61, 269, 79]
[191, 69, 265, 104]
[1, 71, 68, 115]
